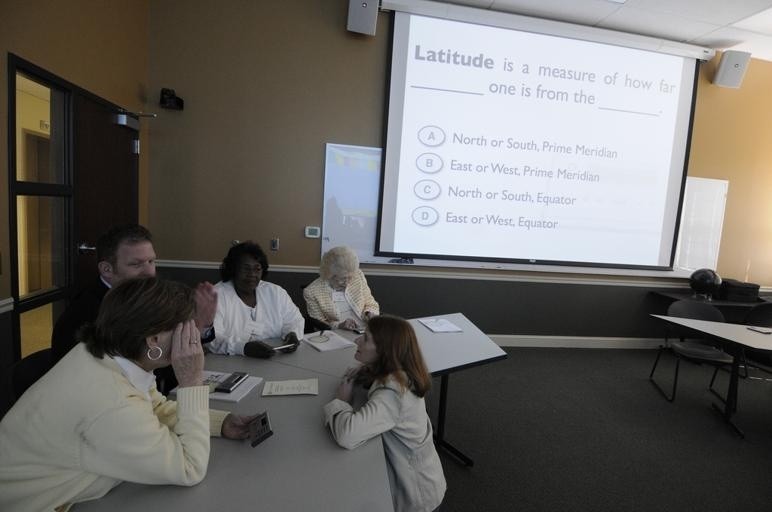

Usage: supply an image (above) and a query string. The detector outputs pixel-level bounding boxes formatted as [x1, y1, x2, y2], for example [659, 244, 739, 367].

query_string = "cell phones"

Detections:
[215, 371, 249, 393]
[353, 328, 365, 334]
[272, 343, 295, 351]
[248, 409, 273, 448]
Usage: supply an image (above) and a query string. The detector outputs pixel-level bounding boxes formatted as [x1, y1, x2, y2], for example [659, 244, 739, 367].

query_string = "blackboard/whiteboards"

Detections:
[320, 142, 729, 282]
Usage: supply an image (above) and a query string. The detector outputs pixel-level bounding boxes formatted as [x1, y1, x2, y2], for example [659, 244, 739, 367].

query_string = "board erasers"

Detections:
[389, 258, 414, 265]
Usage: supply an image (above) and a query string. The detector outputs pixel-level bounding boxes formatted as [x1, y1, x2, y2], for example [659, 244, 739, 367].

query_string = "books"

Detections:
[169, 369, 264, 403]
[302, 328, 357, 353]
[417, 318, 462, 334]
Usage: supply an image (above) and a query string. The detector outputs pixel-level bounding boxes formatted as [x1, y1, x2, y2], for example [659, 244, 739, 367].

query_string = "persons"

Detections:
[323, 312, 449, 511]
[50, 224, 178, 398]
[202, 238, 305, 357]
[0, 274, 264, 512]
[301, 246, 381, 333]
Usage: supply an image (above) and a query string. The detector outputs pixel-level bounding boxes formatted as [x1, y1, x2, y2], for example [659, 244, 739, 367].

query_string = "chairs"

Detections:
[7, 351, 58, 407]
[650, 299, 740, 412]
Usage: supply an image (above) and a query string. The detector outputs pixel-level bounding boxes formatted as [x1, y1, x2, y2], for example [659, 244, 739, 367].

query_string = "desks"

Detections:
[651, 314, 772, 444]
[72, 327, 400, 512]
[652, 288, 770, 325]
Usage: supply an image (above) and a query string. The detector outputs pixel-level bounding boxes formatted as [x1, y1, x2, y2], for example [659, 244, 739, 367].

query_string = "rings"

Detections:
[189, 339, 199, 344]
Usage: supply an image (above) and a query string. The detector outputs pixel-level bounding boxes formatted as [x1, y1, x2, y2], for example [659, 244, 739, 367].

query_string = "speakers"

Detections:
[346, 0, 379, 37]
[712, 50, 752, 89]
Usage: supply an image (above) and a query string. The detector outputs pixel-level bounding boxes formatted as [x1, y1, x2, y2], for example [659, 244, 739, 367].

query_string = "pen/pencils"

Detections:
[482, 265, 503, 268]
